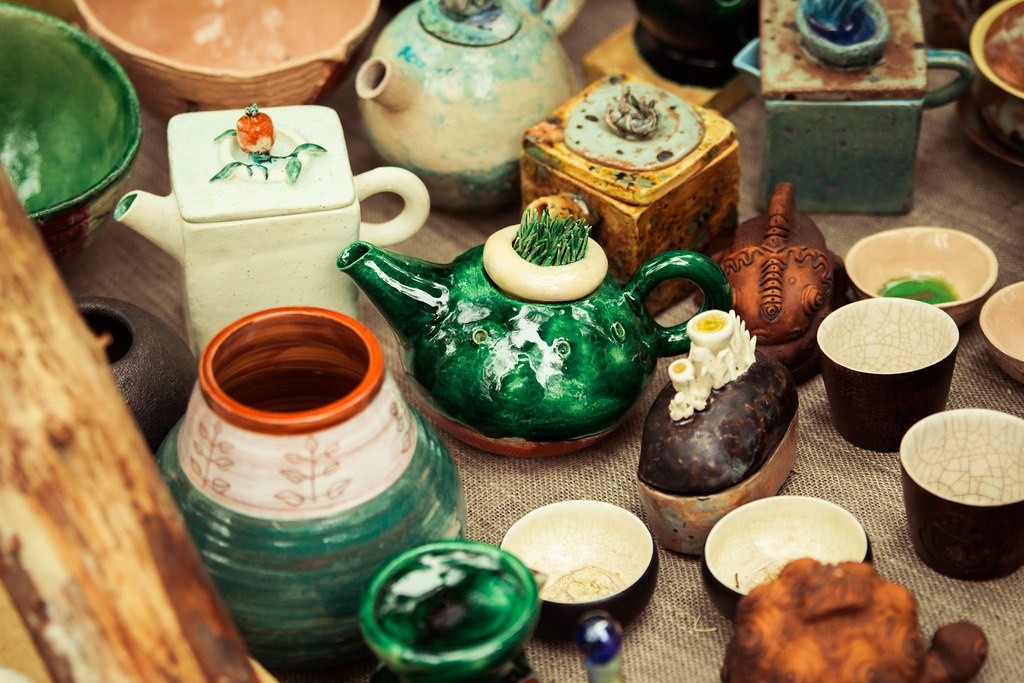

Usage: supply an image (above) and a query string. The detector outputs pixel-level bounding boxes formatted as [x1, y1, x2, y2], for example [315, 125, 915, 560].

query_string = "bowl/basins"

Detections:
[74, 296, 199, 454]
[703, 495, 870, 618]
[500, 499, 659, 633]
[844, 226, 998, 330]
[0, 0, 141, 260]
[73, 0, 380, 119]
[978, 280, 1024, 384]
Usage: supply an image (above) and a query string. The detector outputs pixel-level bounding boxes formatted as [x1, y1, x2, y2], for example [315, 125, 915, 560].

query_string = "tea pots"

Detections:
[356, 0, 587, 217]
[337, 194, 733, 441]
[732, 0, 977, 216]
[110, 105, 430, 365]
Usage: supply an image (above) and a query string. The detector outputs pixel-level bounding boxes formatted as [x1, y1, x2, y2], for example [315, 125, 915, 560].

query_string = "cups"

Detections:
[899, 408, 1024, 580]
[631, 0, 759, 88]
[959, 65, 1024, 168]
[817, 297, 960, 453]
[957, 0, 1024, 151]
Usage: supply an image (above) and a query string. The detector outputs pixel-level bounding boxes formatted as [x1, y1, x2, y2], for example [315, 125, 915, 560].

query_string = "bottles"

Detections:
[355, 540, 543, 683]
[154, 306, 469, 670]
[579, 608, 624, 683]
